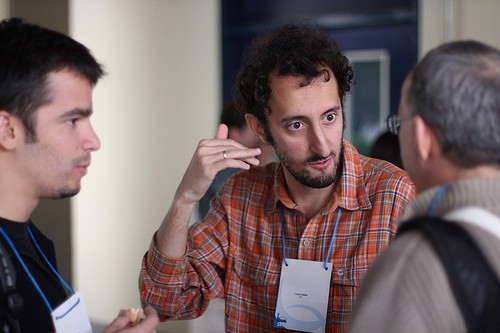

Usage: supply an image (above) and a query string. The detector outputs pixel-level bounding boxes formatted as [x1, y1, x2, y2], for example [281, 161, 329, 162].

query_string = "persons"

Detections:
[138, 20, 500, 333]
[0, 17, 160, 333]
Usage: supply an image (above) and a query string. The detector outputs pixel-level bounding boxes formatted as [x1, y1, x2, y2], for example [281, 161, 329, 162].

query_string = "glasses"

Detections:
[387, 113, 411, 135]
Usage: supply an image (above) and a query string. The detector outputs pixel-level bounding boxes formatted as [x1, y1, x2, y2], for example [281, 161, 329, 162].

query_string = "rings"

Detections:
[223, 151, 227, 159]
